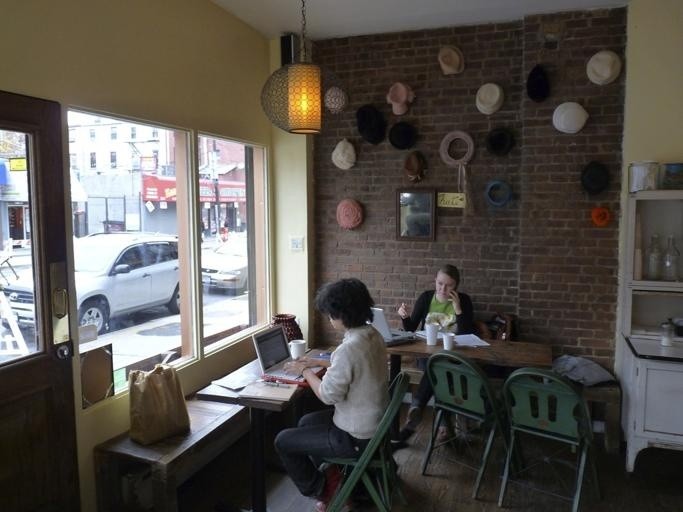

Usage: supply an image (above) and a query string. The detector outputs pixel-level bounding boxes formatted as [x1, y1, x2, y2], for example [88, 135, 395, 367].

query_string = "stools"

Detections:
[582, 382, 622, 458]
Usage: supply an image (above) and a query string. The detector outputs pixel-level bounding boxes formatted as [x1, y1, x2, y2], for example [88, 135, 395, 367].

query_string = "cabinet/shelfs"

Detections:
[614, 189, 683, 473]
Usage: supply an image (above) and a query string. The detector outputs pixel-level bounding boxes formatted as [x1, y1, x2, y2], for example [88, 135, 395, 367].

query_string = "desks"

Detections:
[387, 338, 553, 449]
[94, 399, 252, 512]
[197, 349, 327, 512]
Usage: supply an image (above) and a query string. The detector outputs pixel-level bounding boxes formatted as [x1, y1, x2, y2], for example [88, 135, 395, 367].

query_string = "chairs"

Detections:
[323, 371, 411, 512]
[418, 350, 517, 499]
[498, 367, 602, 512]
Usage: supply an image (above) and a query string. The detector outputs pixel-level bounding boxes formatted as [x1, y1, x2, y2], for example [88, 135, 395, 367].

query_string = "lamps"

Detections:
[260, 1, 351, 135]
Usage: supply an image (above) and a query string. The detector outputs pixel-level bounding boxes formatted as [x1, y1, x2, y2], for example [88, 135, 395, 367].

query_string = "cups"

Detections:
[425, 323, 440, 346]
[288, 339, 307, 360]
[442, 333, 455, 351]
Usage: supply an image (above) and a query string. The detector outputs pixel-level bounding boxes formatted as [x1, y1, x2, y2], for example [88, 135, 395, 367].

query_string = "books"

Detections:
[239, 382, 298, 402]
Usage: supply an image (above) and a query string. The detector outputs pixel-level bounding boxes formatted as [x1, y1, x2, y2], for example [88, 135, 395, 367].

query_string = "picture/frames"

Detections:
[396, 187, 437, 241]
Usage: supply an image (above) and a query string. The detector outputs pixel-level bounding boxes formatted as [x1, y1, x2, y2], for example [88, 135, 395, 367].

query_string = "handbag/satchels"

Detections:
[128, 366, 190, 446]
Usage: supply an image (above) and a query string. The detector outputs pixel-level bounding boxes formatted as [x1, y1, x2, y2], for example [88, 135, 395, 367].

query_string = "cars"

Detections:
[199, 229, 249, 295]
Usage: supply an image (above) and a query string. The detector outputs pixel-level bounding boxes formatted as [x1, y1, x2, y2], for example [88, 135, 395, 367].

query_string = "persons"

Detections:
[398, 264, 473, 441]
[275, 278, 388, 512]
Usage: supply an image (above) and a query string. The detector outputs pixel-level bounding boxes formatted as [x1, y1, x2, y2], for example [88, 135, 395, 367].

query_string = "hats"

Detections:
[389, 122, 416, 150]
[526, 64, 549, 102]
[404, 151, 428, 183]
[331, 104, 386, 230]
[585, 50, 621, 86]
[551, 102, 589, 134]
[580, 160, 608, 195]
[385, 81, 415, 115]
[474, 82, 504, 115]
[484, 127, 512, 156]
[438, 45, 464, 75]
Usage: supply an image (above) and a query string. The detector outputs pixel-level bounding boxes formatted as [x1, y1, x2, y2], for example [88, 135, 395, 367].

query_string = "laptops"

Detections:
[252, 324, 303, 381]
[368, 307, 418, 345]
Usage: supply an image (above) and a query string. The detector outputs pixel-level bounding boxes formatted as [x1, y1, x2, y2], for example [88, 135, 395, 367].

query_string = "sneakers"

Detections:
[319, 471, 342, 505]
[438, 426, 448, 441]
[405, 406, 423, 429]
[312, 499, 352, 512]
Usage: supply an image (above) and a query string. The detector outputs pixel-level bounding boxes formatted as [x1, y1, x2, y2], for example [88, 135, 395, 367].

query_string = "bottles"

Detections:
[644, 233, 661, 280]
[661, 235, 680, 281]
[660, 322, 674, 347]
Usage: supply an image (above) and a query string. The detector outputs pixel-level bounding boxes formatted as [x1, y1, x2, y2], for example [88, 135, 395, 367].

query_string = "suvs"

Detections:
[0, 230, 190, 341]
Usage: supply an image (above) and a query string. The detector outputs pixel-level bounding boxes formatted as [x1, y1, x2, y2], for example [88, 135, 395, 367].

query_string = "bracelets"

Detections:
[302, 366, 310, 374]
